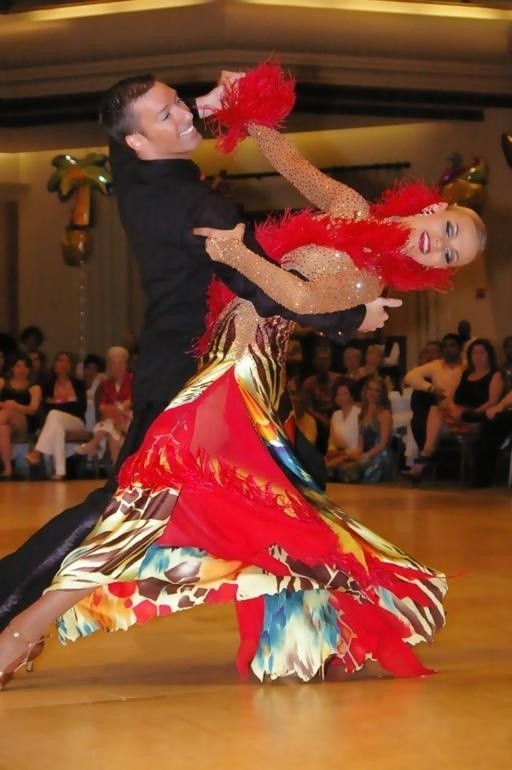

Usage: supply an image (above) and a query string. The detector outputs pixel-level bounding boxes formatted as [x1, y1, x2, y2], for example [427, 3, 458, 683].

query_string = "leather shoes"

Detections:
[402, 470, 421, 483]
[414, 450, 440, 464]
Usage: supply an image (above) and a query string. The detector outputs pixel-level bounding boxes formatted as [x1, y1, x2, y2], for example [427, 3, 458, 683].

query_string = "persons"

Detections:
[1, 326, 134, 481]
[0, 70, 399, 631]
[278, 321, 512, 488]
[0, 54, 487, 686]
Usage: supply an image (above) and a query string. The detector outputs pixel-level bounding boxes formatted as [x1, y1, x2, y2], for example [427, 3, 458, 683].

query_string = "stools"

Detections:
[391, 432, 512, 489]
[10, 430, 112, 480]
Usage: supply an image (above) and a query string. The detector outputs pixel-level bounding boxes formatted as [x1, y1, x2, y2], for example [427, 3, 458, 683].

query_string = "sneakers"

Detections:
[462, 410, 481, 423]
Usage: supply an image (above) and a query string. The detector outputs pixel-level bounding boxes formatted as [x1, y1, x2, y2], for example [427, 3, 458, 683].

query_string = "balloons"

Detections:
[61, 231, 91, 262]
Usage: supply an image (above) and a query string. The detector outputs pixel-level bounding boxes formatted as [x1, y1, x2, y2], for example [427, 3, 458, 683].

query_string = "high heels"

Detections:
[0, 623, 50, 688]
